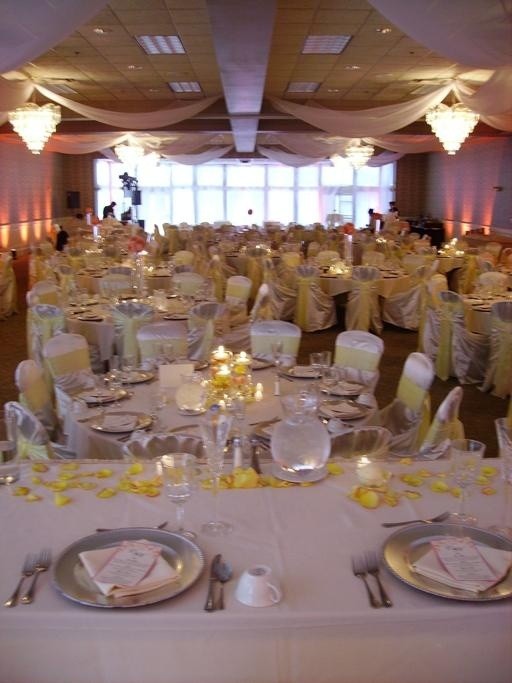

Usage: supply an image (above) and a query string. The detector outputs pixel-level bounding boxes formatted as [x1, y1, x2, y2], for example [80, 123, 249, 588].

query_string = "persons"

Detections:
[368, 208, 386, 230]
[103, 200, 116, 216]
[388, 200, 400, 215]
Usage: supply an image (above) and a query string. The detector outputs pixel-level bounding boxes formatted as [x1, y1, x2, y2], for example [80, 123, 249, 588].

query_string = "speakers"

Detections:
[132, 191, 140, 205]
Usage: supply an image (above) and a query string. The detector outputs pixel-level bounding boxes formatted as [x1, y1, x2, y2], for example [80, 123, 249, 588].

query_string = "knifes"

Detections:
[202, 552, 223, 612]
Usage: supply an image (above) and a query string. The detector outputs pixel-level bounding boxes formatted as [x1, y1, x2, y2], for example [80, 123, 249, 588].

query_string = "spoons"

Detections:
[214, 563, 231, 612]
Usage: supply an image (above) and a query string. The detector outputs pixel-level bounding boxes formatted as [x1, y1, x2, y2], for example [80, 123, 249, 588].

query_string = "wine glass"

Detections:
[159, 448, 202, 543]
[444, 436, 487, 527]
[195, 407, 241, 537]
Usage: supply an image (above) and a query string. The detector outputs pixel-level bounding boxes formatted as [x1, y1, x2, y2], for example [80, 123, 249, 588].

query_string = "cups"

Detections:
[356, 454, 391, 490]
[0, 441, 21, 485]
[493, 417, 511, 484]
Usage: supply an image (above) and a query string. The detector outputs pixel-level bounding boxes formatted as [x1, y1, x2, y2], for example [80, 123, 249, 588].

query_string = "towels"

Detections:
[407, 540, 511, 591]
[76, 536, 181, 598]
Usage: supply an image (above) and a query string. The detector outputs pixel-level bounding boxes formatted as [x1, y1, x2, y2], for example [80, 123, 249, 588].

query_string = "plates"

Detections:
[66, 292, 206, 324]
[76, 358, 378, 433]
[268, 461, 331, 482]
[461, 290, 503, 313]
[321, 264, 408, 282]
[47, 527, 203, 611]
[69, 262, 175, 278]
[382, 524, 512, 608]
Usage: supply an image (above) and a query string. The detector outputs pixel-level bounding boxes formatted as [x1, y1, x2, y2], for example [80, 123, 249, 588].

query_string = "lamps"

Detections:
[343, 134, 378, 176]
[4, 75, 63, 157]
[423, 79, 483, 157]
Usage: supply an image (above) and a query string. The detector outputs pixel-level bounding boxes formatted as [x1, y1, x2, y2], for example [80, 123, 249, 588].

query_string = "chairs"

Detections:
[2, 210, 512, 459]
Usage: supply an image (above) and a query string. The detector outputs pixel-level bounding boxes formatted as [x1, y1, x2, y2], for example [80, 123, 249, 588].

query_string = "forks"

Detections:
[2, 552, 39, 608]
[380, 510, 451, 529]
[362, 551, 394, 606]
[351, 551, 382, 609]
[95, 520, 168, 533]
[21, 549, 52, 604]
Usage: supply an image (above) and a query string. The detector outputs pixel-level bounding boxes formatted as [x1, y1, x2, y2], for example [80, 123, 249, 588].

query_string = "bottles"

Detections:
[233, 563, 284, 610]
[268, 390, 329, 472]
[248, 439, 262, 473]
[232, 438, 242, 471]
